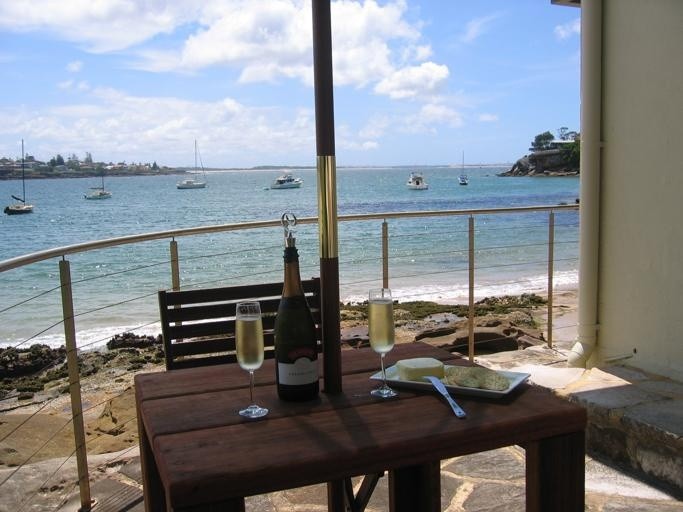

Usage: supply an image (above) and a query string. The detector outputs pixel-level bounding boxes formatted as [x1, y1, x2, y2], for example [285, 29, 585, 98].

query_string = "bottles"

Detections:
[275, 212, 320, 401]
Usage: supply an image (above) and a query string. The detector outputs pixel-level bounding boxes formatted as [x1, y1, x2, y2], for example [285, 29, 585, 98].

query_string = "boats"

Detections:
[83, 189, 112, 201]
[270, 172, 302, 191]
[406, 170, 428, 191]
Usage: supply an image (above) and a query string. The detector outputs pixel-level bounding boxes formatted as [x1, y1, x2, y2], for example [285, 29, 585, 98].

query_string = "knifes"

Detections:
[423, 372, 468, 420]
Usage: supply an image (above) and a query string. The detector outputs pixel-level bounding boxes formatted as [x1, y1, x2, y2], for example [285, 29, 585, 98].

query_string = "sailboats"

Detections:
[3, 139, 35, 215]
[455, 149, 469, 186]
[175, 138, 208, 191]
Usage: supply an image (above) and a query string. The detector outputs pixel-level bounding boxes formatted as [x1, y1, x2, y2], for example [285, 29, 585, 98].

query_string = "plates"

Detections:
[371, 361, 531, 402]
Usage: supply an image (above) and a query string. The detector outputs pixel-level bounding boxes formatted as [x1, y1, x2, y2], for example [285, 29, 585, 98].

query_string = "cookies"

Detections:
[445, 366, 509, 392]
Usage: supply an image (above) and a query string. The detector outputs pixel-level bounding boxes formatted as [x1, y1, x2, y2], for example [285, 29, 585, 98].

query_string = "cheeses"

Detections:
[397, 357, 445, 383]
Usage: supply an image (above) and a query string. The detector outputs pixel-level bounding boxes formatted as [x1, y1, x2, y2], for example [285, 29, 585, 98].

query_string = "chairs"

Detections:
[153, 276, 387, 511]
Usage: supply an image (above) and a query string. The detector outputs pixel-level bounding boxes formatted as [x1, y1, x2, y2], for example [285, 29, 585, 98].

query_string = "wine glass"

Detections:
[367, 287, 397, 401]
[232, 299, 270, 419]
